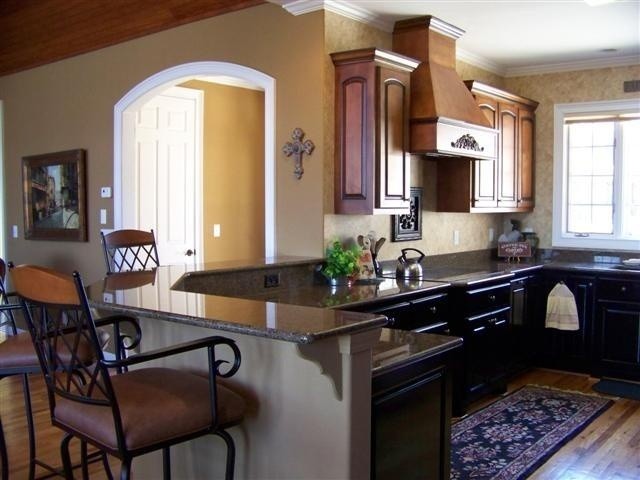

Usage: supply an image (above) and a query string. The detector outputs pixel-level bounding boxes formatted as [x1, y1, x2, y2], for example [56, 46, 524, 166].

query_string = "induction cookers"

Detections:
[380, 267, 505, 282]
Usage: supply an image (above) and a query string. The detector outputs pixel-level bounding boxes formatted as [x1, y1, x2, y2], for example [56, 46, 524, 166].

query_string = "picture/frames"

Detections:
[20, 148, 89, 242]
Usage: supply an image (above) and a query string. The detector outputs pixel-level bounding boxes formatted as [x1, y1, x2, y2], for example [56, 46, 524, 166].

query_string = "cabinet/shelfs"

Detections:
[593, 272, 639, 378]
[372, 343, 460, 478]
[372, 290, 452, 334]
[437, 78, 539, 216]
[459, 282, 517, 400]
[546, 268, 593, 375]
[507, 268, 547, 386]
[329, 48, 421, 215]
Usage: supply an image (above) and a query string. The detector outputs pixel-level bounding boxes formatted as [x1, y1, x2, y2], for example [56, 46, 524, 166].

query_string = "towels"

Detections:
[544, 278, 581, 331]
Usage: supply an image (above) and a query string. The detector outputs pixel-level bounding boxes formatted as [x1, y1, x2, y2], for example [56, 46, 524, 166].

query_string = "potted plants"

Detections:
[319, 237, 361, 284]
[322, 285, 353, 307]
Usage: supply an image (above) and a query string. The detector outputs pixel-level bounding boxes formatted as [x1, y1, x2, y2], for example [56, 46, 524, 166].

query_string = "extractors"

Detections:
[391, 15, 497, 162]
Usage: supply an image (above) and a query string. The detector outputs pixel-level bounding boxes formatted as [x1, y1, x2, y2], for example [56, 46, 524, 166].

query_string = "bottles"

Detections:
[372, 254, 379, 274]
[356, 249, 376, 280]
[521, 232, 536, 263]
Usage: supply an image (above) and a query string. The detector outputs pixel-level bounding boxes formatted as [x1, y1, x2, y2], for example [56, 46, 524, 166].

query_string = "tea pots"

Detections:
[396, 247, 425, 279]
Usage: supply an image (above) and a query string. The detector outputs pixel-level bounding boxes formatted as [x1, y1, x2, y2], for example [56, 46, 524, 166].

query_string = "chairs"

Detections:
[2, 228, 248, 477]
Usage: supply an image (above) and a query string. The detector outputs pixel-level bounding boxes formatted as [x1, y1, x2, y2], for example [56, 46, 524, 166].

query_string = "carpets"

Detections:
[592, 376, 639, 400]
[445, 385, 615, 479]
